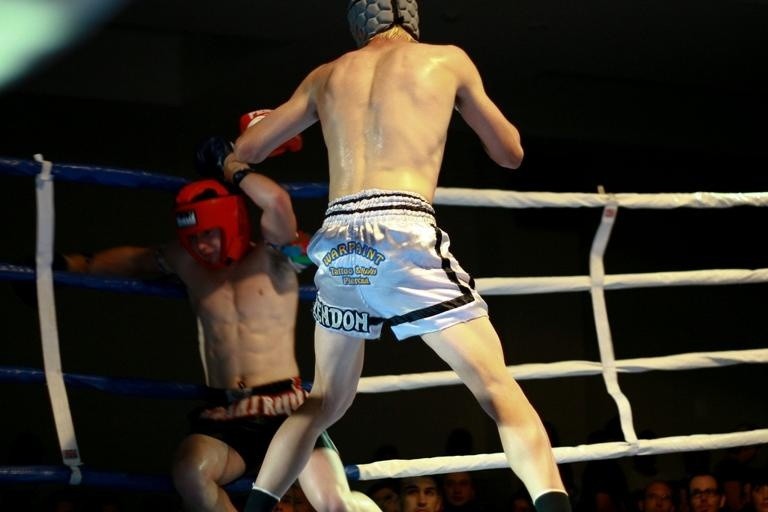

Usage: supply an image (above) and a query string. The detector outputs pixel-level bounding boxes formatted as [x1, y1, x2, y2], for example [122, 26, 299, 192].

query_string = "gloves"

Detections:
[195, 134, 254, 189]
[9, 251, 69, 307]
[240, 107, 304, 161]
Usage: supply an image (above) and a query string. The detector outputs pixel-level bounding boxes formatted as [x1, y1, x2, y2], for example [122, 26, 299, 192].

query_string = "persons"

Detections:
[45, 125, 386, 511]
[503, 415, 768, 509]
[234, 3, 578, 508]
[281, 428, 484, 508]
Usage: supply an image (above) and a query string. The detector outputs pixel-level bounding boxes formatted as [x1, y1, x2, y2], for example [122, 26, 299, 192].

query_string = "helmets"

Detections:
[345, 0, 420, 48]
[175, 179, 252, 275]
